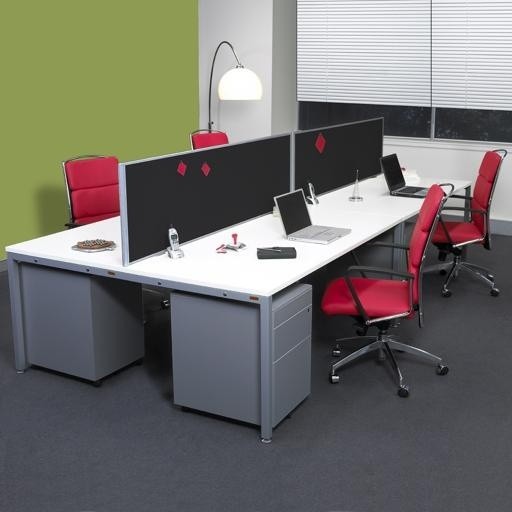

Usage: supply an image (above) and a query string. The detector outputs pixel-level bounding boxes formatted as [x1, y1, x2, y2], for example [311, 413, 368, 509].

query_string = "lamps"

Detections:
[208, 41, 263, 133]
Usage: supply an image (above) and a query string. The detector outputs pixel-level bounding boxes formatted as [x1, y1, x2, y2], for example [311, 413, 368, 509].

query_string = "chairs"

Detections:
[190, 129, 228, 150]
[431, 150, 507, 297]
[61, 155, 120, 229]
[320, 183, 454, 397]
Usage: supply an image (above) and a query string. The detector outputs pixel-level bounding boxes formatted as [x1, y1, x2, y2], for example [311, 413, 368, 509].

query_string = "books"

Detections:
[257, 247, 296, 259]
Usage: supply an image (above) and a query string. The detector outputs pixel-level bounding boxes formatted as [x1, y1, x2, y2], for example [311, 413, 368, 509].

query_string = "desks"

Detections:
[4, 172, 471, 444]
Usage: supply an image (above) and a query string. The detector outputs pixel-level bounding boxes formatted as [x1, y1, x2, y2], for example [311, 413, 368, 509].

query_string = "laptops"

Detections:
[378, 152, 431, 198]
[273, 188, 351, 246]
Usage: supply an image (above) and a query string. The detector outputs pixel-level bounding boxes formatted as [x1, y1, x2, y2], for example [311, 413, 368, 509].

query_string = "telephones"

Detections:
[167, 223, 183, 260]
[306, 182, 319, 205]
